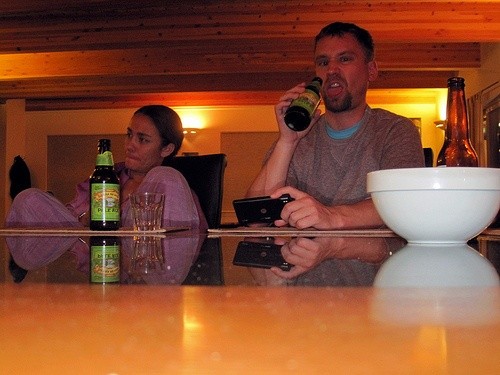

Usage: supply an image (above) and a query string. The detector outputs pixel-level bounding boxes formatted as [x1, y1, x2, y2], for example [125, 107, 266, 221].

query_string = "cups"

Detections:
[129, 233, 165, 274]
[129, 191, 166, 230]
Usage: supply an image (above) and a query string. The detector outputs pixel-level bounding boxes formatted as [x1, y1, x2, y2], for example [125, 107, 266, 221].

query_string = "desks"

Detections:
[0, 225, 500, 375]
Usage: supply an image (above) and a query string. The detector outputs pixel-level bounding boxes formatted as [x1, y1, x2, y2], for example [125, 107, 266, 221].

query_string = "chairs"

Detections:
[114, 154, 228, 228]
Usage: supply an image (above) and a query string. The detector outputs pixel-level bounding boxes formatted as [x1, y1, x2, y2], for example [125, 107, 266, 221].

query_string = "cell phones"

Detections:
[233, 241, 291, 271]
[233, 194, 292, 226]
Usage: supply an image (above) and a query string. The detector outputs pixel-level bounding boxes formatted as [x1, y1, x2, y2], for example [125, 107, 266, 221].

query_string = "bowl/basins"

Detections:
[372, 244, 499, 324]
[366, 166, 500, 243]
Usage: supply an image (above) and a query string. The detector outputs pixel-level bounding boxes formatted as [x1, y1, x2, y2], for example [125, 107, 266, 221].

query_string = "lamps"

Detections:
[181, 114, 205, 143]
[433, 96, 447, 130]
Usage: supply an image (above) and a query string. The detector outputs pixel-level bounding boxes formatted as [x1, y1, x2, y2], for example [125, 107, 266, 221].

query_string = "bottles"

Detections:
[89, 139, 121, 231]
[284, 77, 323, 132]
[436, 77, 479, 167]
[89, 237, 122, 286]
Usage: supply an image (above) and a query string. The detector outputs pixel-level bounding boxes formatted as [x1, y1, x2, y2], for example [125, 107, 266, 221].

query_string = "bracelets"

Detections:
[79, 212, 87, 218]
[77, 237, 89, 250]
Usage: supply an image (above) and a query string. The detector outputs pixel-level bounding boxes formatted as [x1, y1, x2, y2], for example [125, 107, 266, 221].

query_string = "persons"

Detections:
[5, 232, 207, 286]
[7, 104, 211, 230]
[243, 236, 408, 288]
[244, 22, 426, 230]
